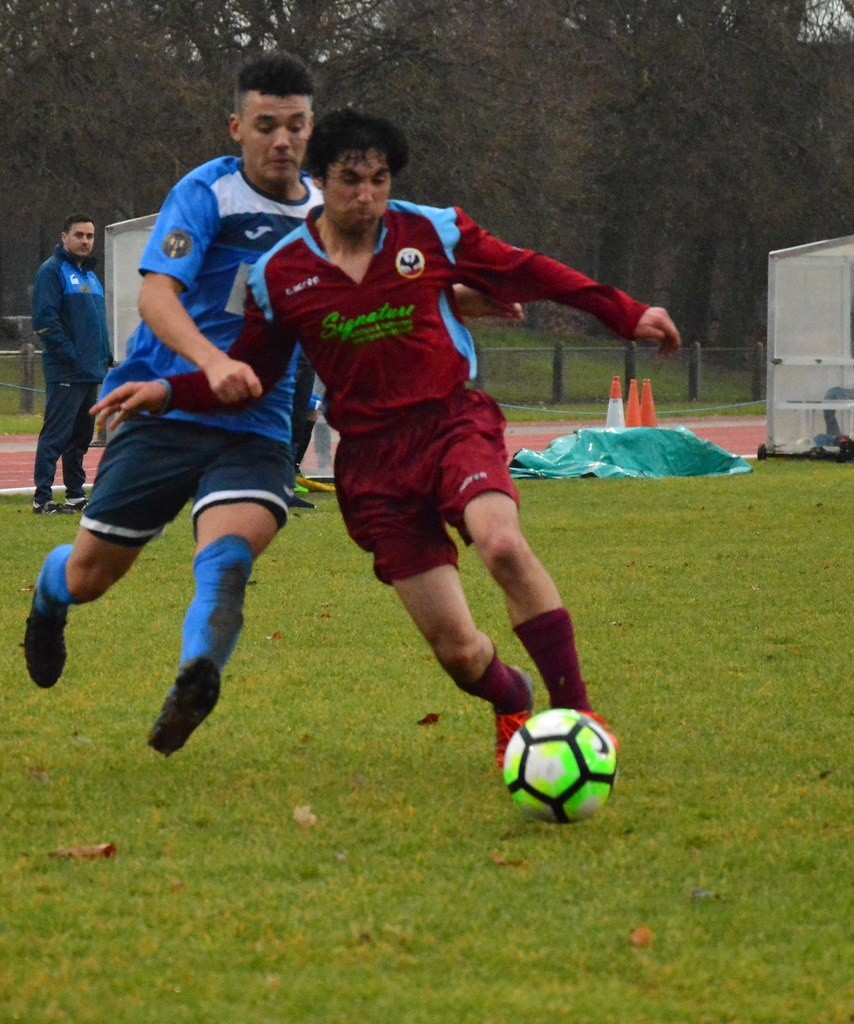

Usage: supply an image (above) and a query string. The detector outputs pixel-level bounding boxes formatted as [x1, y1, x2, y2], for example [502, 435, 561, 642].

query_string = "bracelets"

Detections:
[154, 376, 173, 416]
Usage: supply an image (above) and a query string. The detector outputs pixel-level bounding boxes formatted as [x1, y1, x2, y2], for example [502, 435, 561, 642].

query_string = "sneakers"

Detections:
[32, 498, 90, 516]
[579, 706, 620, 755]
[494, 666, 538, 771]
[24, 588, 67, 690]
[148, 656, 221, 758]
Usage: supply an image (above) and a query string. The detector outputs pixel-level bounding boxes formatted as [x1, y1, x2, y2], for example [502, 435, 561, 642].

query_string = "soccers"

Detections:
[501, 707, 620, 827]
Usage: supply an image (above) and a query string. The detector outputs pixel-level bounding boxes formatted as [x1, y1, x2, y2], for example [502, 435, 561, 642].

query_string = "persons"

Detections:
[23, 49, 525, 757]
[29, 211, 114, 516]
[89, 109, 682, 769]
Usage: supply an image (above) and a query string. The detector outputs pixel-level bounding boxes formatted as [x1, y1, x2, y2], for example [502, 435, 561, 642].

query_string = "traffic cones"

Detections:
[641, 377, 658, 427]
[627, 379, 641, 427]
[605, 375, 625, 428]
